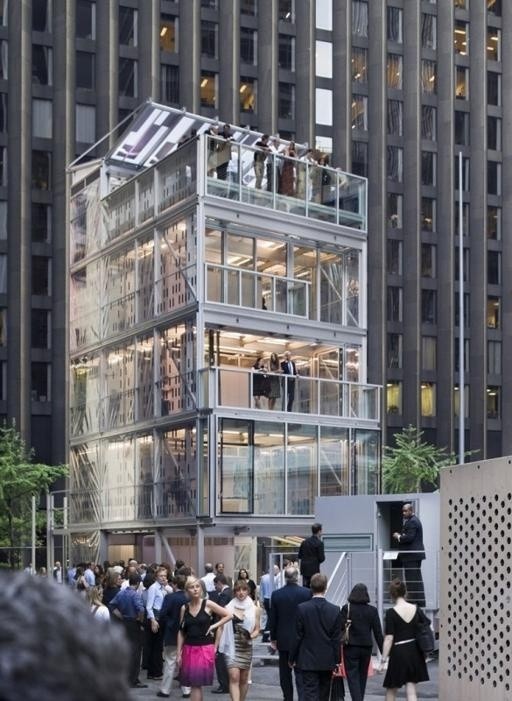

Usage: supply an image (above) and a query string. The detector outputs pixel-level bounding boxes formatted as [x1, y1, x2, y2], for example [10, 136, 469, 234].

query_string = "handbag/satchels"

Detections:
[238, 625, 250, 640]
[339, 602, 352, 646]
[414, 606, 434, 652]
[77, 575, 85, 590]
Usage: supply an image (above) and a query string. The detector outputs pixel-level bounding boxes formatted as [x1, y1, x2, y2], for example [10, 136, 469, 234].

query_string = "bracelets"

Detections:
[380, 659, 387, 664]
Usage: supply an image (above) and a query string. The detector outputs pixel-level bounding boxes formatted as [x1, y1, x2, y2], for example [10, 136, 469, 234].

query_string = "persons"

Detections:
[393, 504, 426, 607]
[207, 123, 349, 209]
[281, 351, 299, 412]
[377, 577, 431, 701]
[0, 524, 340, 700]
[252, 357, 268, 410]
[266, 353, 284, 410]
[340, 584, 382, 701]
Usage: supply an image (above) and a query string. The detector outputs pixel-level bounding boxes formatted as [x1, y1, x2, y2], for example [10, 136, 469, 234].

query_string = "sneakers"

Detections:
[153, 673, 163, 680]
[157, 692, 169, 697]
[135, 678, 148, 688]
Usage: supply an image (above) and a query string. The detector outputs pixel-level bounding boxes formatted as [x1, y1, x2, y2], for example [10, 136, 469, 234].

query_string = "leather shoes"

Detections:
[211, 687, 229, 693]
